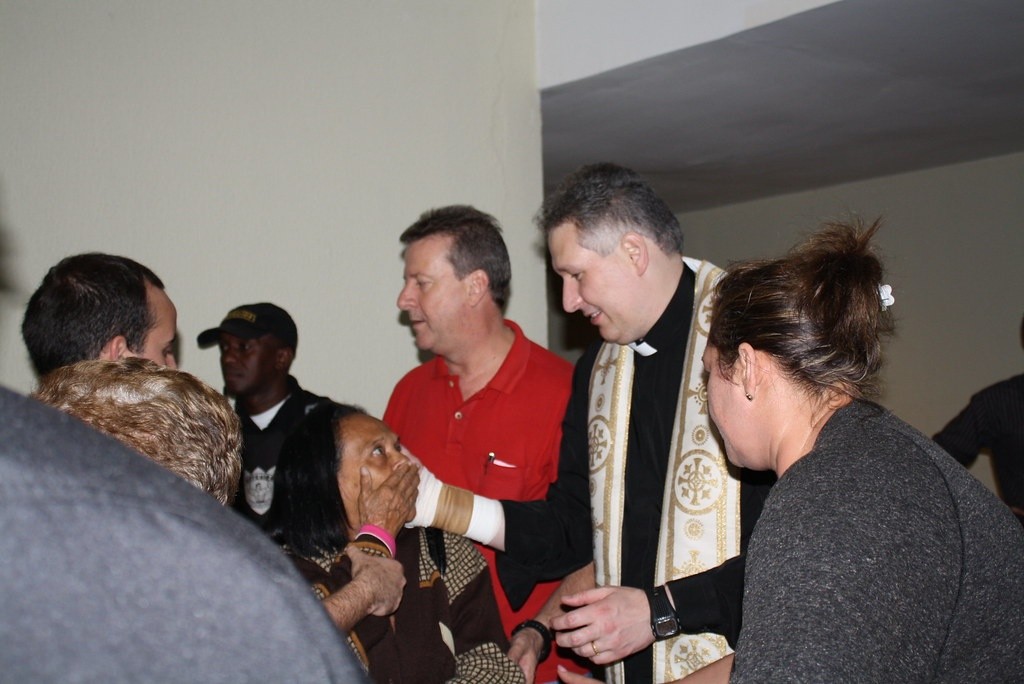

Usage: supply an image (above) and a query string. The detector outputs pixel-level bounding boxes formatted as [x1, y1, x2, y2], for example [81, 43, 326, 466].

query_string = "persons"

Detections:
[263, 402, 527, 683]
[23, 357, 244, 505]
[398, 164, 778, 684]
[557, 233, 1024, 684]
[932, 316, 1024, 517]
[21, 253, 407, 636]
[0, 387, 367, 684]
[196, 302, 320, 528]
[381, 205, 596, 684]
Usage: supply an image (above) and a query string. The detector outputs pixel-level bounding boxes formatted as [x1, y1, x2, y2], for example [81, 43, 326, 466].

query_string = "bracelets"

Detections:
[511, 620, 551, 663]
[359, 525, 396, 558]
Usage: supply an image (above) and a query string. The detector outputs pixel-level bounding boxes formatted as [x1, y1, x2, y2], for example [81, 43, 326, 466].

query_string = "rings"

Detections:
[592, 641, 599, 655]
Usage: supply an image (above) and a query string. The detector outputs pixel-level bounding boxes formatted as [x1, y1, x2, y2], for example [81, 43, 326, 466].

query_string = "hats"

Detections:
[196, 302, 298, 353]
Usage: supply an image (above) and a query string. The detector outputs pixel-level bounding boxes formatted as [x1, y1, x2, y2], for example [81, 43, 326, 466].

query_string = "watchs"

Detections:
[646, 585, 681, 641]
